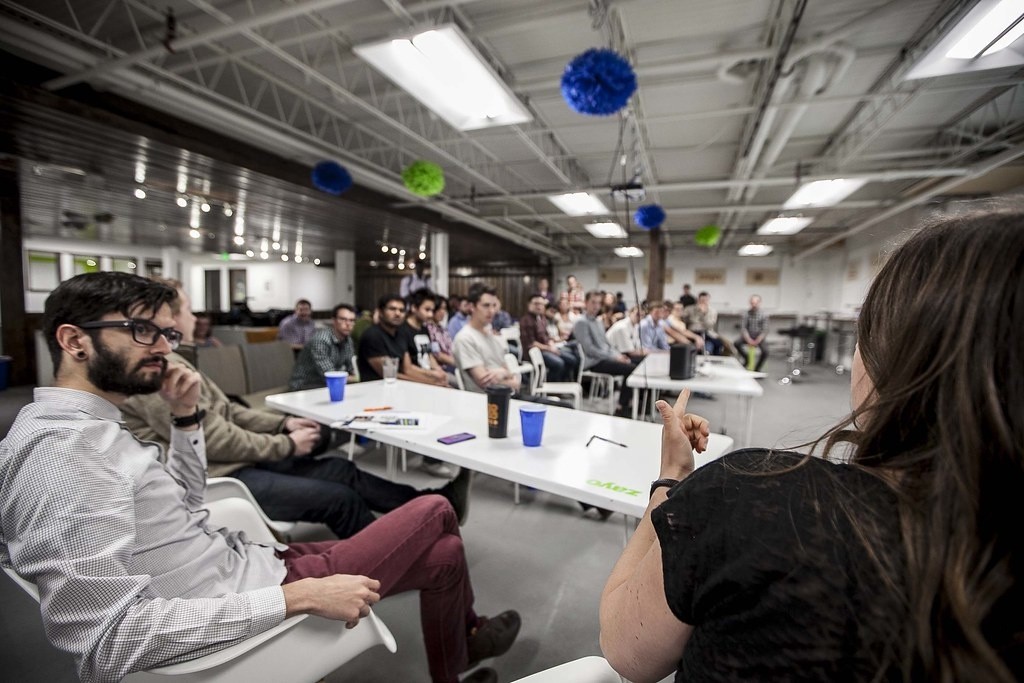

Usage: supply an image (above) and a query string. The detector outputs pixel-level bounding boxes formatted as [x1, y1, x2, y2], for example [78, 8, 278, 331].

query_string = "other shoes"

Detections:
[614, 407, 633, 419]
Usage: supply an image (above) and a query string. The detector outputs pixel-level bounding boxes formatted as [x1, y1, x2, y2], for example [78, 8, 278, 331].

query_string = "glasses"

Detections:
[389, 306, 405, 313]
[88, 318, 183, 351]
[426, 307, 433, 312]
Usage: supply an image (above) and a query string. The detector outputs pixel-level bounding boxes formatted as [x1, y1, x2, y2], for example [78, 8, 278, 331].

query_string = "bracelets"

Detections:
[169, 406, 207, 428]
[650, 479, 680, 497]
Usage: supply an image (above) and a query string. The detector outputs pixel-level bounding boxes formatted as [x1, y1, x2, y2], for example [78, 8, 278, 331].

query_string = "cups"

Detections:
[323, 371, 348, 401]
[485, 383, 512, 438]
[382, 358, 399, 384]
[520, 405, 547, 447]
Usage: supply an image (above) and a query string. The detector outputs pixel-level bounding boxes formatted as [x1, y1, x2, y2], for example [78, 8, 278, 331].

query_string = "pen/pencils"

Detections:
[364, 407, 393, 410]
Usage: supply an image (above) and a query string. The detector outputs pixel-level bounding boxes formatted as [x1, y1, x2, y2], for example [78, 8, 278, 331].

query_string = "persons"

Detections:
[120, 278, 474, 541]
[598, 205, 1024, 683]
[278, 260, 738, 478]
[0, 272, 523, 683]
[735, 294, 770, 370]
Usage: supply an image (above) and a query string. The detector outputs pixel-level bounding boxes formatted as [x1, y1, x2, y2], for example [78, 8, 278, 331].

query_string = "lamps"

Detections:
[545, 187, 644, 258]
[897, 0, 1023, 83]
[740, 176, 866, 258]
[351, 20, 533, 131]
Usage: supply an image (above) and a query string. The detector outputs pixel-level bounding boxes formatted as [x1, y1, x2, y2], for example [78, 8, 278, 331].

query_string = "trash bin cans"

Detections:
[803, 329, 825, 362]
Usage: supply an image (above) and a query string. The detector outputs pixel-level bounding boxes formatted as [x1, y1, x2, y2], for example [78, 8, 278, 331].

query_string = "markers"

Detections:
[380, 419, 418, 426]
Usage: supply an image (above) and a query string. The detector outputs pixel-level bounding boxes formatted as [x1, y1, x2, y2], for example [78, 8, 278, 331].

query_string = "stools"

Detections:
[744, 343, 767, 372]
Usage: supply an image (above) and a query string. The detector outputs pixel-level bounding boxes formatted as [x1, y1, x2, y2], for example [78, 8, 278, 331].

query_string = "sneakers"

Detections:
[424, 466, 470, 525]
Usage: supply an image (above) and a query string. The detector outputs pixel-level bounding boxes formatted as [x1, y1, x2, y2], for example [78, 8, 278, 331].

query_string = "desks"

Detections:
[263, 378, 733, 548]
[626, 353, 763, 446]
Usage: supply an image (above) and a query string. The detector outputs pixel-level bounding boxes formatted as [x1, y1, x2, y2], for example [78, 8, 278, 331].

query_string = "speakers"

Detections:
[669, 343, 697, 380]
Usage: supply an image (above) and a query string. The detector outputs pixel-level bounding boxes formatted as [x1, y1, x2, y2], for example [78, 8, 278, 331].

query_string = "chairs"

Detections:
[346, 327, 624, 504]
[195, 339, 296, 409]
[0, 497, 399, 683]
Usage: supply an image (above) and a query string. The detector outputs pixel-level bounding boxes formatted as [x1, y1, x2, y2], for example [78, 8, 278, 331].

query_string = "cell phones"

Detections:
[438, 432, 476, 445]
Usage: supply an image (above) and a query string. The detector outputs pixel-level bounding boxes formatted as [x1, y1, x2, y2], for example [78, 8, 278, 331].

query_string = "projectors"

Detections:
[612, 185, 646, 204]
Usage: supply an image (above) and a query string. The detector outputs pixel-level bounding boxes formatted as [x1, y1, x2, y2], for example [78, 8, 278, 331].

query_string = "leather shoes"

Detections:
[459, 609, 522, 674]
[460, 668, 498, 682]
